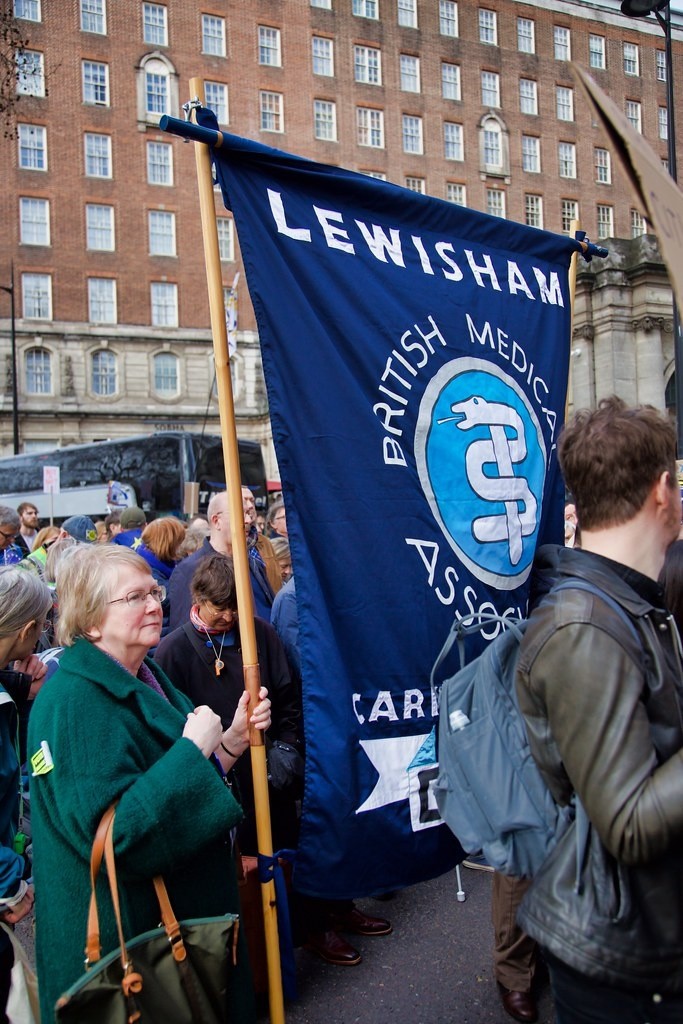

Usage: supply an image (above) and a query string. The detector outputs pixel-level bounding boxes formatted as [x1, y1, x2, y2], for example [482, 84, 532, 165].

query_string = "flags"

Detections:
[195, 105, 578, 901]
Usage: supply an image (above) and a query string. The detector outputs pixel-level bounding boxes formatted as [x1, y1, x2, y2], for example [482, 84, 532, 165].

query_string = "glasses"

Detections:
[35, 620, 51, 632]
[0, 531, 19, 539]
[200, 593, 238, 618]
[105, 585, 167, 607]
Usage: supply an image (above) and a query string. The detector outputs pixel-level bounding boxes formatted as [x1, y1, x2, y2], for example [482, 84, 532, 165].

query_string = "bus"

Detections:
[0, 429, 270, 532]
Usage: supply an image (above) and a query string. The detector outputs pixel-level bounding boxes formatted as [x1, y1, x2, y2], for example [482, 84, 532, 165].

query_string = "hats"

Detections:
[62, 515, 98, 544]
[120, 507, 146, 529]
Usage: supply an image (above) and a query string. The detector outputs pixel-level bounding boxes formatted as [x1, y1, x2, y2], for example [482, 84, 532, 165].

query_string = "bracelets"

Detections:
[221, 742, 243, 758]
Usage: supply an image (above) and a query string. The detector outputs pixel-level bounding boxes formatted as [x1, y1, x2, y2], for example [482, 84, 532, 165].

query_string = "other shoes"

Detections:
[463, 854, 495, 873]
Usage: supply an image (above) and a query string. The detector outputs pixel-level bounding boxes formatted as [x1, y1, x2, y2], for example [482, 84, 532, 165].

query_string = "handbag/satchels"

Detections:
[55, 803, 240, 1024]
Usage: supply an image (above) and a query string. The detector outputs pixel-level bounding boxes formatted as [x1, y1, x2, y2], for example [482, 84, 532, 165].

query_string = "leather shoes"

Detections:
[326, 907, 392, 935]
[497, 980, 538, 1023]
[312, 931, 362, 965]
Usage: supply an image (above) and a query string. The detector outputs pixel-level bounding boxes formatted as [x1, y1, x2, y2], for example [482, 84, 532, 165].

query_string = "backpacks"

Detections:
[432, 578, 644, 876]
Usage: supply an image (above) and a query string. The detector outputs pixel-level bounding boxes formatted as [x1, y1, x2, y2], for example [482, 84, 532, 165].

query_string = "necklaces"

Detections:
[202, 625, 225, 675]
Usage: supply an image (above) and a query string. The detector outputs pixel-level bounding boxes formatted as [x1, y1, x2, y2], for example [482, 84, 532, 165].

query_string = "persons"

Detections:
[462, 392, 683, 1024]
[0, 485, 391, 1024]
